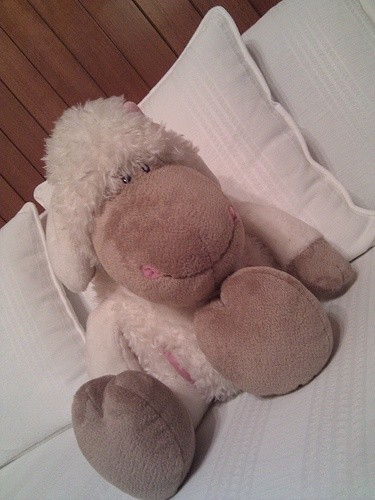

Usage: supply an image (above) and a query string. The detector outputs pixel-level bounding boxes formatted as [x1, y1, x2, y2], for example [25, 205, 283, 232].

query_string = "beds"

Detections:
[0, 241, 373, 500]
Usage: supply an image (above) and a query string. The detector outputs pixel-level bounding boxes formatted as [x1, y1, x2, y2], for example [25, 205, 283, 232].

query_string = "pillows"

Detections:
[30, 6, 375, 303]
[228, 1, 375, 211]
[0, 198, 103, 468]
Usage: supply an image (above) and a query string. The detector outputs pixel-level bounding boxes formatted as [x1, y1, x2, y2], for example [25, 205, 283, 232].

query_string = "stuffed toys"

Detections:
[44, 94, 357, 499]
